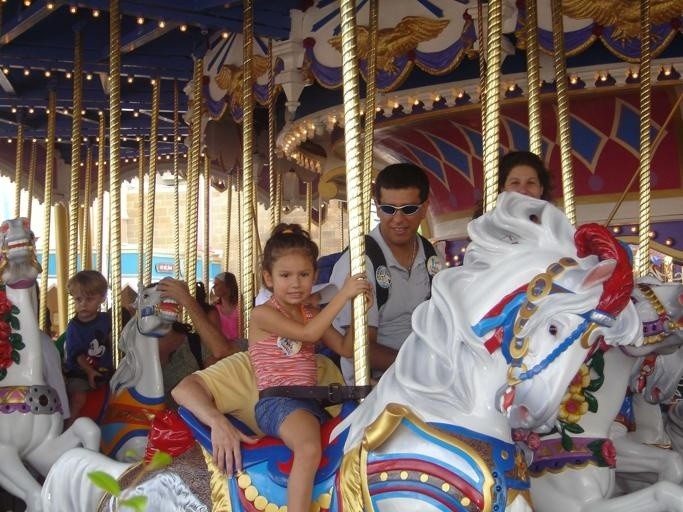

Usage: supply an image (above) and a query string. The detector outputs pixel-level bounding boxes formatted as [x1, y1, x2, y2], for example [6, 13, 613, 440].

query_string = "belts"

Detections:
[257, 383, 372, 401]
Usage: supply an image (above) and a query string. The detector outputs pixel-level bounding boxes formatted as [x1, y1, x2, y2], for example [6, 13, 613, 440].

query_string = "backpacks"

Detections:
[314, 232, 437, 358]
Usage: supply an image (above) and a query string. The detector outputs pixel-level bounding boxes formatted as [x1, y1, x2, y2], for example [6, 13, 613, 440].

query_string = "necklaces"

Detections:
[408, 238, 417, 272]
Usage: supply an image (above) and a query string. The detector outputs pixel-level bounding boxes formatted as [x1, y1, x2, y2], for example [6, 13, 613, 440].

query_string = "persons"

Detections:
[171, 282, 347, 479]
[66, 270, 248, 429]
[472, 150, 550, 219]
[328, 164, 447, 399]
[248, 223, 374, 512]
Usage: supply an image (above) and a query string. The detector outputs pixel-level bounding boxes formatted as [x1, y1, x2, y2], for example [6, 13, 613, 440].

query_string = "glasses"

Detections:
[375, 200, 429, 216]
